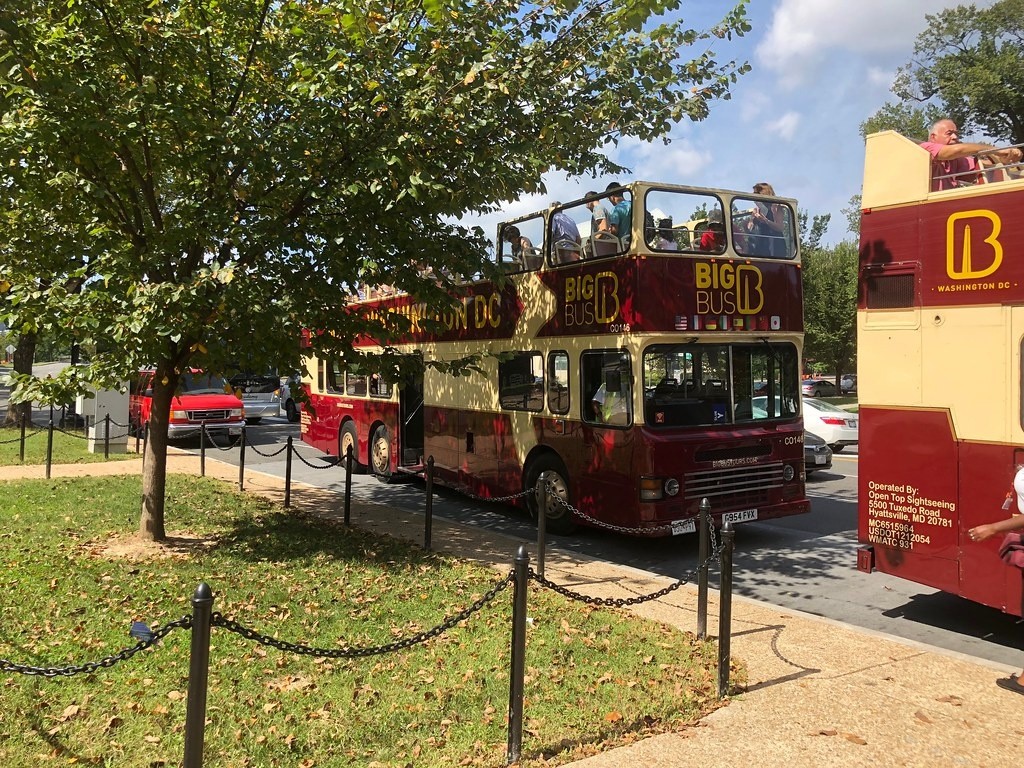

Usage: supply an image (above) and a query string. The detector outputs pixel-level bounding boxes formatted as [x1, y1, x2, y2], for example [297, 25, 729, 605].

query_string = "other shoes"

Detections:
[996, 673, 1024, 695]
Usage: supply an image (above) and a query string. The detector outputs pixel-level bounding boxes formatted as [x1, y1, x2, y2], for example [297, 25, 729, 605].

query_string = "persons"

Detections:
[741, 183, 788, 258]
[503, 226, 536, 261]
[699, 210, 747, 253]
[592, 382, 631, 424]
[551, 201, 581, 265]
[656, 219, 678, 251]
[585, 182, 656, 253]
[920, 119, 1023, 192]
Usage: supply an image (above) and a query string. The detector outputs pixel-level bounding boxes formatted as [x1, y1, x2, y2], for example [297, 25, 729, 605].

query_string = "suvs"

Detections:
[127, 371, 246, 449]
[840, 374, 857, 394]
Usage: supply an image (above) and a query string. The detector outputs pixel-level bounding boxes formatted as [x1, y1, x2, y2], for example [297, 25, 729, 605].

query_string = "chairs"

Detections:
[502, 254, 523, 276]
[554, 238, 584, 264]
[591, 231, 622, 258]
[654, 378, 677, 396]
[583, 243, 593, 259]
[521, 247, 544, 272]
[621, 234, 631, 252]
[704, 379, 725, 394]
[969, 152, 1024, 184]
[677, 378, 695, 394]
[690, 236, 701, 252]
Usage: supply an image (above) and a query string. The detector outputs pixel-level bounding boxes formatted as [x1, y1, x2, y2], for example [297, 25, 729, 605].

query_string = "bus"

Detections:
[204, 326, 281, 422]
[299, 180, 813, 540]
[853, 129, 1024, 620]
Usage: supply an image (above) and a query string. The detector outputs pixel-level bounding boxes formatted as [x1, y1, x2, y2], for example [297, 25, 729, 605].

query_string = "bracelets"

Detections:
[597, 412, 602, 417]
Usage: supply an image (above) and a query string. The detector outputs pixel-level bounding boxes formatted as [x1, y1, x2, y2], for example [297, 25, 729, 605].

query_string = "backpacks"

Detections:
[629, 199, 656, 243]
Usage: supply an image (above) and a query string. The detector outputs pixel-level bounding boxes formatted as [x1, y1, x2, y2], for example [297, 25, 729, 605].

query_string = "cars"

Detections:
[281, 368, 301, 422]
[802, 380, 837, 398]
[752, 395, 858, 452]
[752, 406, 833, 475]
